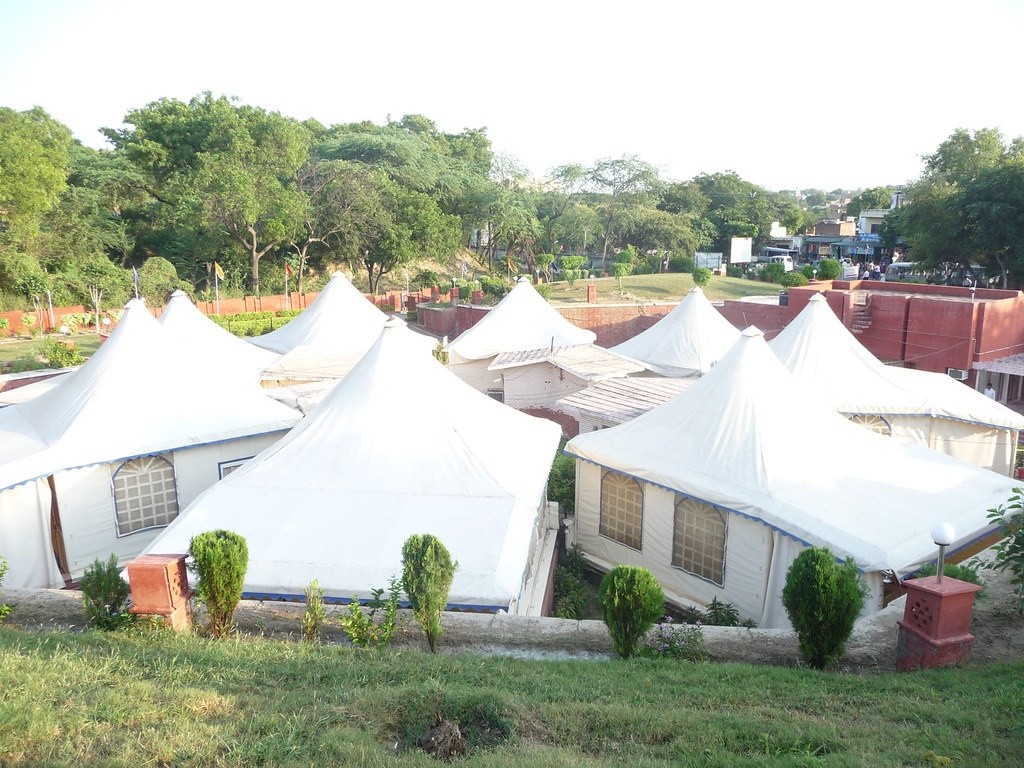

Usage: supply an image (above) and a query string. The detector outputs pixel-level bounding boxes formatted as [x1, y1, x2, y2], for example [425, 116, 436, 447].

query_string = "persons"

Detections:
[832, 256, 906, 281]
[925, 267, 972, 286]
[984, 383, 997, 400]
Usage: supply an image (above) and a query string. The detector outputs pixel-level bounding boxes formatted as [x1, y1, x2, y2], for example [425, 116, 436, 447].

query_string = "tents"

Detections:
[0, 272, 1024, 628]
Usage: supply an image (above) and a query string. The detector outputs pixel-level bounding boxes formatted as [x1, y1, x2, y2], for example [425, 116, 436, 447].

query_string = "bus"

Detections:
[757, 246, 799, 265]
[884, 261, 990, 288]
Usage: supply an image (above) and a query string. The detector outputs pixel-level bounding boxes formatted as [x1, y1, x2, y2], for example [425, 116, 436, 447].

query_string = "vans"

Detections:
[766, 254, 794, 272]
